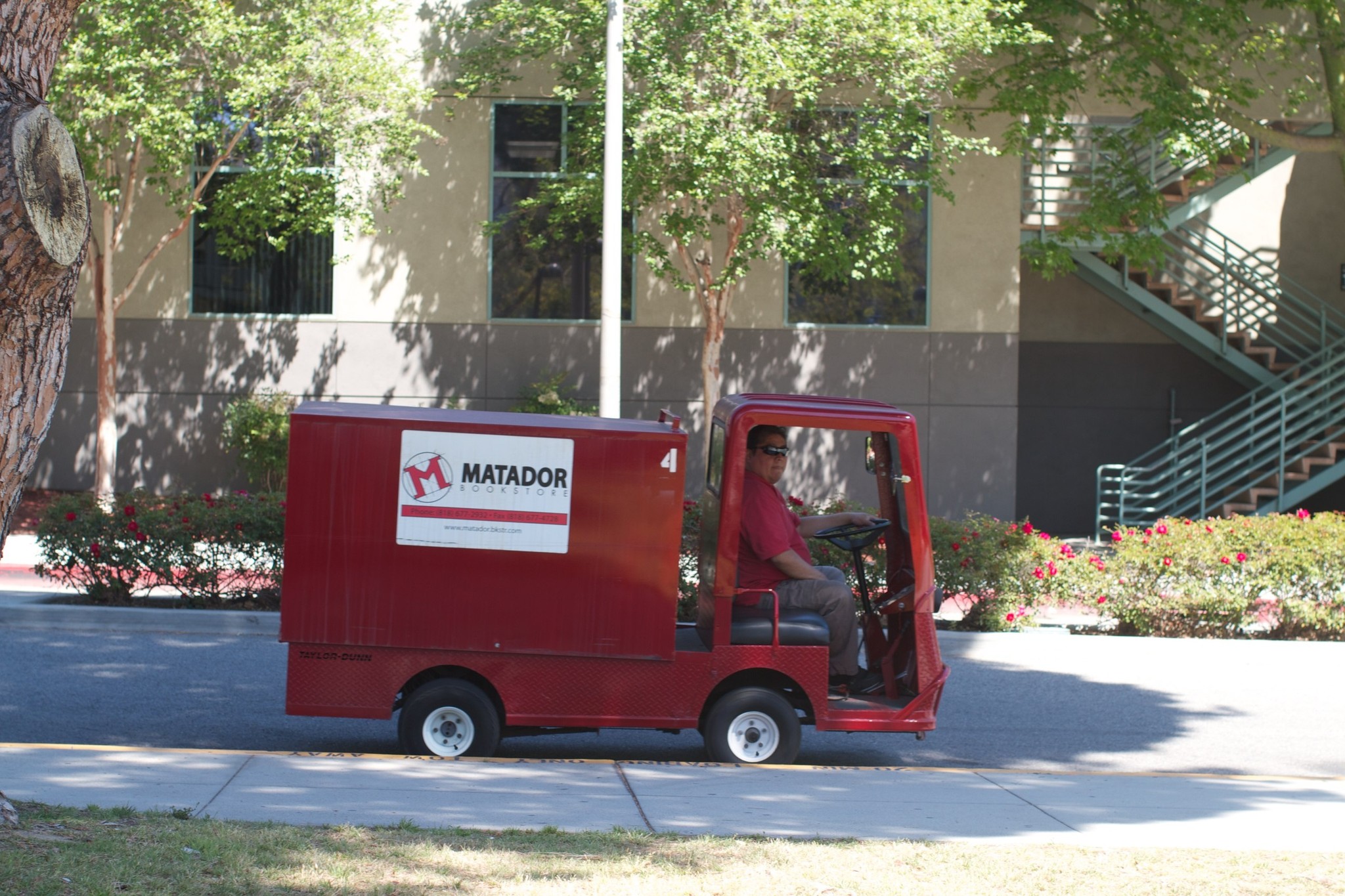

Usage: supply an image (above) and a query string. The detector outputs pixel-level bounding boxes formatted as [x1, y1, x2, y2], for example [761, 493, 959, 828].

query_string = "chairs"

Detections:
[731, 585, 831, 644]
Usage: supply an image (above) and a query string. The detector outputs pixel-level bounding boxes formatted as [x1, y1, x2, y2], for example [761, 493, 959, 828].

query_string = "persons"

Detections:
[735, 424, 889, 693]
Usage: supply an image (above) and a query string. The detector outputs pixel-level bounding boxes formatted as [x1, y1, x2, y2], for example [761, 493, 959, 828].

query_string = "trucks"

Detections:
[276, 390, 951, 764]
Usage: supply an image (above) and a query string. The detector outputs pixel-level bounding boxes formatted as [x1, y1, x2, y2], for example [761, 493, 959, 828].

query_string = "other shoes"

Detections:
[828, 665, 883, 694]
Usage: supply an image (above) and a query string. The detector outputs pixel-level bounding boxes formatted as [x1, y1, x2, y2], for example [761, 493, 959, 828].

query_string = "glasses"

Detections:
[747, 445, 789, 457]
